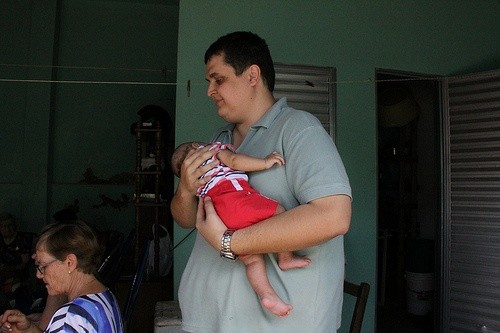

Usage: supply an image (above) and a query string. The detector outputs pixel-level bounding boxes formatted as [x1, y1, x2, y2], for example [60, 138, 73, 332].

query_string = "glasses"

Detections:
[35, 259, 59, 273]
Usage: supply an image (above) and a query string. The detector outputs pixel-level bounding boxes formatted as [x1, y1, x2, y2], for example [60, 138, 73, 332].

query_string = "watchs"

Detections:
[220, 229, 238, 259]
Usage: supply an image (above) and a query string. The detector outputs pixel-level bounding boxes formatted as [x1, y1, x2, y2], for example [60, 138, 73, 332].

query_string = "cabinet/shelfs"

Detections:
[132, 119, 161, 291]
[379, 118, 417, 308]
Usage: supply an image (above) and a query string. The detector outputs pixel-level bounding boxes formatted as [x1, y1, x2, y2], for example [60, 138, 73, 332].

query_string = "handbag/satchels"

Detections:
[149, 223, 173, 276]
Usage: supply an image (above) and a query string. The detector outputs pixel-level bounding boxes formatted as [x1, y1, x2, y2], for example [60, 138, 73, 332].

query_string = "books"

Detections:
[134, 193, 162, 202]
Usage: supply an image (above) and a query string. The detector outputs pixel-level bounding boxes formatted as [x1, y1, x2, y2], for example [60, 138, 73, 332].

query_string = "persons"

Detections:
[171, 31, 353, 333]
[0, 212, 125, 333]
[170, 142, 312, 319]
